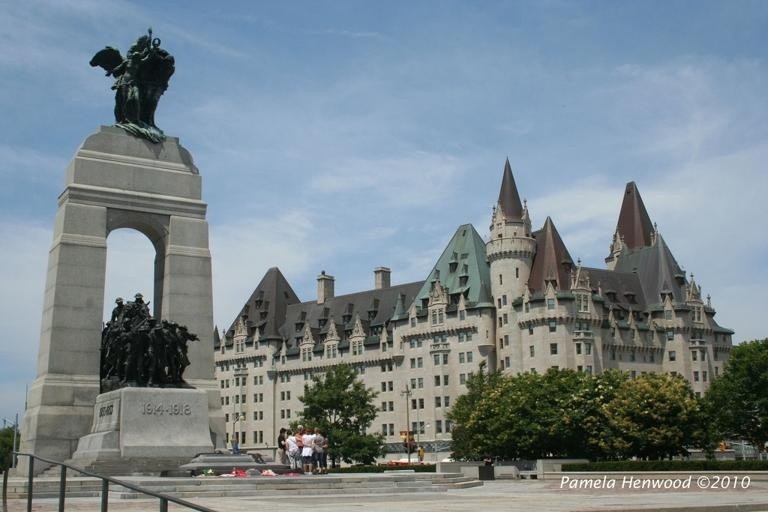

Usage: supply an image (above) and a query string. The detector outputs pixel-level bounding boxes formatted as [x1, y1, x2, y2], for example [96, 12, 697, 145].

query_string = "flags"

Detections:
[399, 430, 418, 455]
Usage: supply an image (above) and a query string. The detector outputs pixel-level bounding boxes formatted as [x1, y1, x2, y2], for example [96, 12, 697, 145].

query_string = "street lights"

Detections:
[416, 422, 430, 447]
[231, 415, 245, 441]
[399, 384, 412, 464]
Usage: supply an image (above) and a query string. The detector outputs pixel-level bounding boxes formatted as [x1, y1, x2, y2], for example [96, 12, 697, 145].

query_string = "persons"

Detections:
[719, 439, 726, 455]
[482, 453, 493, 480]
[99, 292, 202, 389]
[276, 424, 329, 475]
[103, 26, 156, 126]
[126, 32, 149, 60]
[417, 446, 425, 462]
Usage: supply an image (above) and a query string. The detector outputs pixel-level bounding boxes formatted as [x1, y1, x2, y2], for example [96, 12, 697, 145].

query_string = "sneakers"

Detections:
[304, 469, 328, 475]
